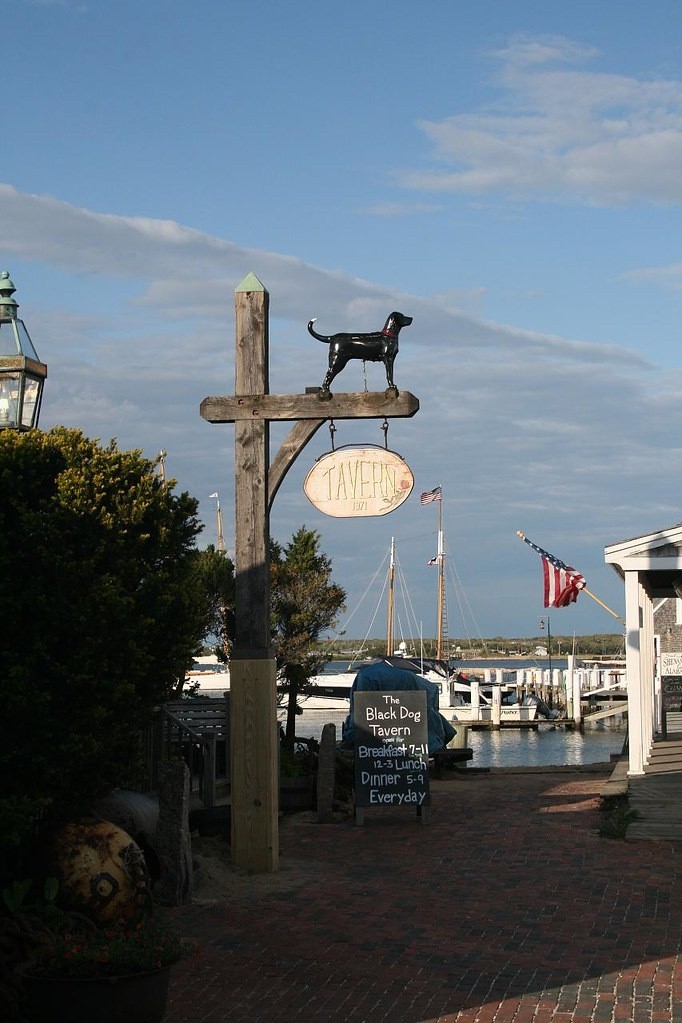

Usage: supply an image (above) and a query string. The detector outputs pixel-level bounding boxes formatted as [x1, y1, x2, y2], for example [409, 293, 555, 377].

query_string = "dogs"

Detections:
[307, 311, 412, 392]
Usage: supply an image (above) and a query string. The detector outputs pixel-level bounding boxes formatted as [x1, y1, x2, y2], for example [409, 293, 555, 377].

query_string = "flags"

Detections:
[427, 557, 438, 565]
[523, 536, 587, 608]
[209, 491, 219, 498]
[421, 485, 442, 506]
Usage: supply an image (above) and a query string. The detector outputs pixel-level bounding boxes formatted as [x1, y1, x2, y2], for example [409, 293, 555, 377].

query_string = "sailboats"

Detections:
[171, 484, 561, 728]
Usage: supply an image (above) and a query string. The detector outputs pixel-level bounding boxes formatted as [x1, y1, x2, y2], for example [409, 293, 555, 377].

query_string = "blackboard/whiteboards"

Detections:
[350, 687, 432, 810]
